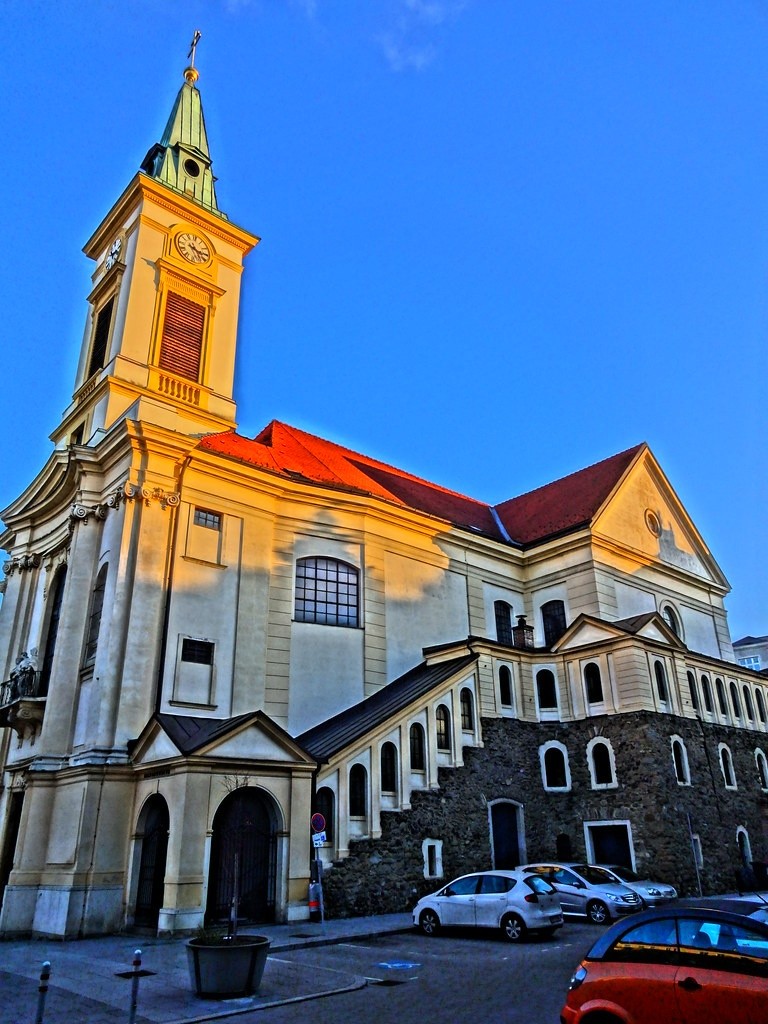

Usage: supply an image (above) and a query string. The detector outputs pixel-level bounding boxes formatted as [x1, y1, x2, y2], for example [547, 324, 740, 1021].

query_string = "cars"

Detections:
[412, 869, 563, 943]
[665, 889, 767, 950]
[514, 862, 644, 924]
[557, 863, 679, 908]
[559, 900, 768, 1024]
